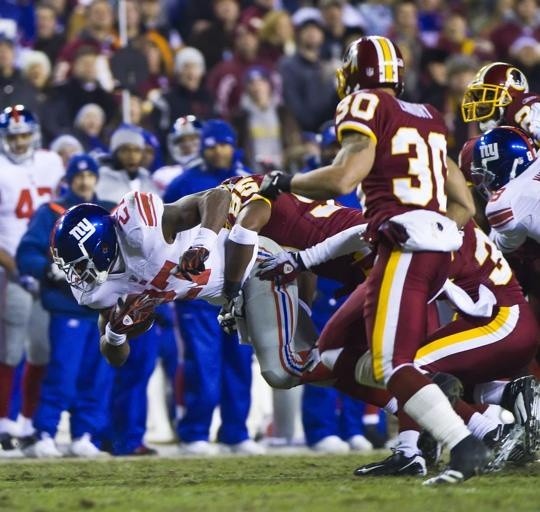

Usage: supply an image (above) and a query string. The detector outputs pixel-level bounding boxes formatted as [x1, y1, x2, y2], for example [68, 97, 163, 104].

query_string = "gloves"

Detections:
[215, 302, 237, 336]
[258, 169, 292, 201]
[179, 244, 209, 282]
[256, 252, 300, 286]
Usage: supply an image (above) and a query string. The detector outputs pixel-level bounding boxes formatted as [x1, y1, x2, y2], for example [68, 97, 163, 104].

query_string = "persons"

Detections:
[1, 0, 540, 485]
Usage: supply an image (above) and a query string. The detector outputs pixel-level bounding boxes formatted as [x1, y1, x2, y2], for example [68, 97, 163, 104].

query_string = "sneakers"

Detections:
[354, 448, 427, 476]
[420, 372, 540, 490]
[314, 435, 349, 450]
[350, 434, 371, 453]
[0, 413, 60, 460]
[128, 445, 159, 458]
[233, 442, 266, 459]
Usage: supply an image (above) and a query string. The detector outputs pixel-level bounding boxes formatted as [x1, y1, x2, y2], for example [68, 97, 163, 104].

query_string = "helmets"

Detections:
[49, 204, 120, 296]
[0, 104, 41, 166]
[166, 114, 205, 163]
[471, 125, 537, 198]
[336, 36, 404, 99]
[460, 61, 530, 127]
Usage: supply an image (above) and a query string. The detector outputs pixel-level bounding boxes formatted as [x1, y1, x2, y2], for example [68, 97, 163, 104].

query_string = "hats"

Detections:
[201, 118, 238, 159]
[321, 126, 338, 149]
[110, 127, 145, 156]
[65, 155, 99, 180]
